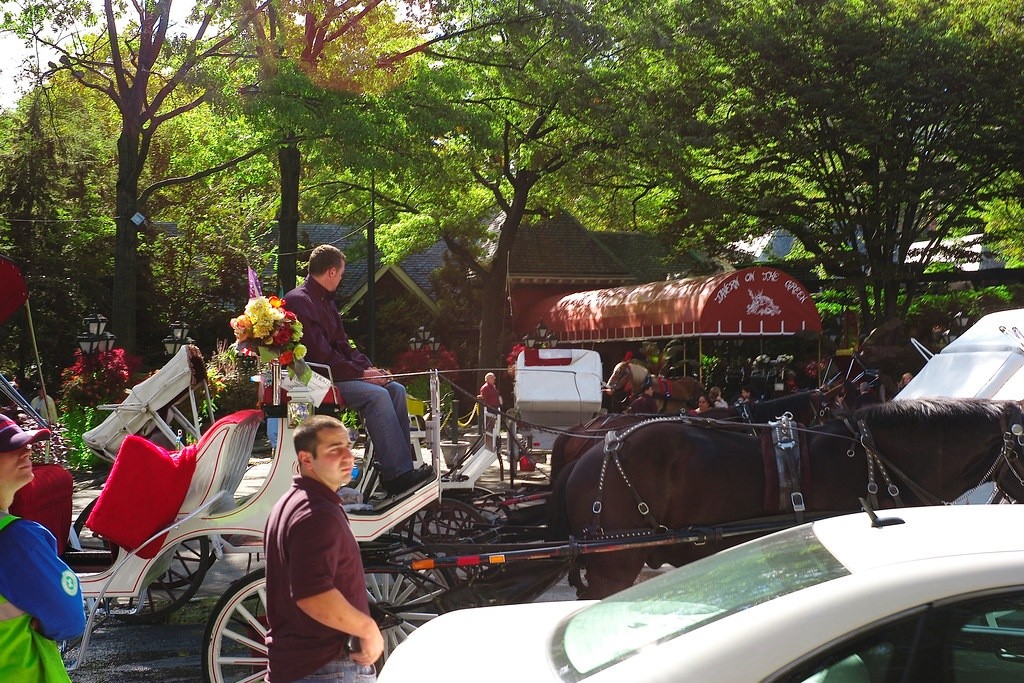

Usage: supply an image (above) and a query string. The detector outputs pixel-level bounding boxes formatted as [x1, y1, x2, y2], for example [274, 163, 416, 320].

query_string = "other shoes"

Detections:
[383, 465, 433, 499]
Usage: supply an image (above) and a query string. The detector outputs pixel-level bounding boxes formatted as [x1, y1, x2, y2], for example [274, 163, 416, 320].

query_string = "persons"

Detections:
[688, 387, 728, 417]
[897, 373, 913, 394]
[31, 382, 57, 425]
[742, 384, 757, 404]
[855, 382, 872, 406]
[480, 372, 503, 426]
[264, 415, 384, 683]
[628, 384, 658, 414]
[0, 413, 87, 683]
[279, 245, 433, 495]
[784, 370, 798, 393]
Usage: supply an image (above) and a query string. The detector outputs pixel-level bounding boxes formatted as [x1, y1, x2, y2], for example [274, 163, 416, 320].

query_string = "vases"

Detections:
[440, 441, 471, 467]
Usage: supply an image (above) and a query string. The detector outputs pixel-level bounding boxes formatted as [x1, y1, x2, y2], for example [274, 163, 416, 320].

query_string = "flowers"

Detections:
[229, 294, 306, 367]
[752, 355, 770, 368]
[777, 355, 793, 369]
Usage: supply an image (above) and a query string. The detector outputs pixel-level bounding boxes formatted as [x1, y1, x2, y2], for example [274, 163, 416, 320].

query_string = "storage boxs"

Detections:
[516, 349, 602, 448]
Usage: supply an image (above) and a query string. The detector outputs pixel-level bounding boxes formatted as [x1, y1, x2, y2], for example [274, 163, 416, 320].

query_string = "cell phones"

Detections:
[347, 635, 361, 654]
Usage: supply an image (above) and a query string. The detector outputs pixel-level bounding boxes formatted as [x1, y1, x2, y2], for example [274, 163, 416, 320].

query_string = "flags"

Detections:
[248, 265, 263, 298]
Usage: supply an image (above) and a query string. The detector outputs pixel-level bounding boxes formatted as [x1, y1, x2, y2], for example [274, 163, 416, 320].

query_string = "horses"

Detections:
[552, 382, 1024, 600]
[605, 361, 707, 413]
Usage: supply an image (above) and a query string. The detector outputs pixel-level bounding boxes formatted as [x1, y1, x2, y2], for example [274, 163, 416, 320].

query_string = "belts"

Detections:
[335, 652, 351, 661]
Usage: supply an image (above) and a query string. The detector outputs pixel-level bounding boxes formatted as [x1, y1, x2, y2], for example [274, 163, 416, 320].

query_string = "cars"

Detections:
[376, 501, 1024, 683]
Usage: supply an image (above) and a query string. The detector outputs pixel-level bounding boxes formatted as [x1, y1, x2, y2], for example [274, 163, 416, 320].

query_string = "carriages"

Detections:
[205, 365, 1024, 683]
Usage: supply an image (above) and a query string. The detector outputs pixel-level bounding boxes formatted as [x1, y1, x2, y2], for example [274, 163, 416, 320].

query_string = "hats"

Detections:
[0, 413, 51, 452]
[33, 382, 47, 392]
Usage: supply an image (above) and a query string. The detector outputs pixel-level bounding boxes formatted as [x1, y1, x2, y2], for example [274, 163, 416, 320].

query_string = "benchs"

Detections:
[258, 358, 347, 420]
[87, 408, 264, 560]
